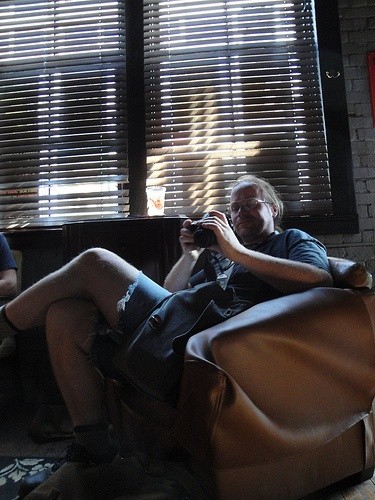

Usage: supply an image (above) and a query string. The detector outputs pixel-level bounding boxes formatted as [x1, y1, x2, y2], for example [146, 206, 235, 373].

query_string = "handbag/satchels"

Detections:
[114, 281, 247, 406]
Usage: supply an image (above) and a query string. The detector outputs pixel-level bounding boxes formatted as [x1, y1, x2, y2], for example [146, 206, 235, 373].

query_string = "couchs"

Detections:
[100, 256, 375, 500]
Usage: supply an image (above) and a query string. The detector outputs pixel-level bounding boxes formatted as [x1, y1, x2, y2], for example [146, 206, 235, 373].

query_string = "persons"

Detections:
[0, 231, 20, 298]
[1, 173, 334, 462]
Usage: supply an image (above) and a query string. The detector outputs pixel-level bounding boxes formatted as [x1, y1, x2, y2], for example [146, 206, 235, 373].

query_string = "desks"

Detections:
[63, 215, 231, 286]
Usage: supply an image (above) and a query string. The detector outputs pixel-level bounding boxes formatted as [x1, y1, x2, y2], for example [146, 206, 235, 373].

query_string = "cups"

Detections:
[145, 185, 167, 217]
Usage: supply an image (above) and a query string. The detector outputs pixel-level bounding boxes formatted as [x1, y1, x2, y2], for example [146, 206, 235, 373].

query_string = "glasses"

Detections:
[226, 199, 274, 213]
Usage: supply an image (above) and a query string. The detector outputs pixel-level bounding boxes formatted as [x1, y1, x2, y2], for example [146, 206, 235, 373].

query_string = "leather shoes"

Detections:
[18, 441, 119, 496]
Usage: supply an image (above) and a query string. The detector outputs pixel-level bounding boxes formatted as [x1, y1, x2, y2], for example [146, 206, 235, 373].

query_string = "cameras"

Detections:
[188, 213, 218, 248]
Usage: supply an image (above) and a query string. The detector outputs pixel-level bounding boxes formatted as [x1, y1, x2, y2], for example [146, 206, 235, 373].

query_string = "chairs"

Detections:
[1, 227, 69, 408]
